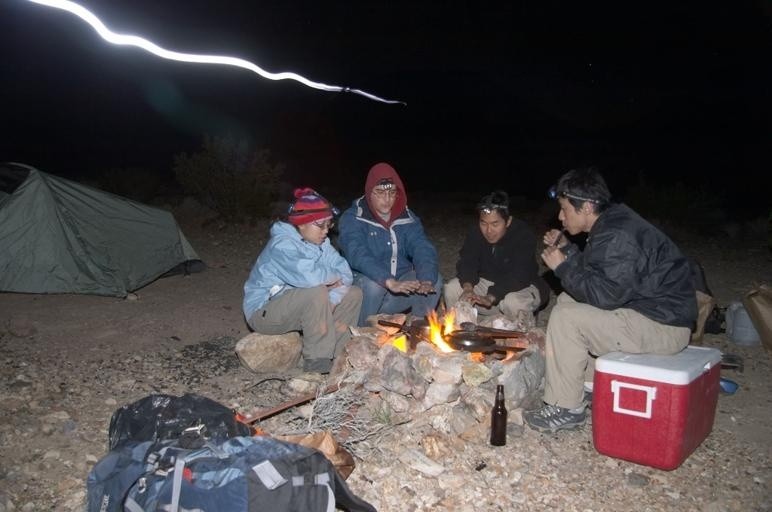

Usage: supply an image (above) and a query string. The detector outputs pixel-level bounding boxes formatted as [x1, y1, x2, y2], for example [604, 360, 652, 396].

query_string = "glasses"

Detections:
[315, 221, 335, 229]
[372, 190, 399, 198]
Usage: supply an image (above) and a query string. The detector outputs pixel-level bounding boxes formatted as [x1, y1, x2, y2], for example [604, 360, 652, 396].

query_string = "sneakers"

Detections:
[522, 389, 593, 432]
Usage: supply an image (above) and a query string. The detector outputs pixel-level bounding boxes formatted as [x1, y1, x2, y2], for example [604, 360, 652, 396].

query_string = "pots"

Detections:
[378, 315, 530, 354]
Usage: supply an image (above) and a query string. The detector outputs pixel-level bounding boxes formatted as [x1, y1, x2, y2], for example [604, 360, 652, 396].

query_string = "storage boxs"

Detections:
[592, 345, 721, 471]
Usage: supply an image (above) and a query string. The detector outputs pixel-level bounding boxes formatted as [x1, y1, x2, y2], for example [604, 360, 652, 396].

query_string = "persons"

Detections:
[443, 189, 553, 334]
[520, 165, 699, 435]
[334, 162, 444, 333]
[241, 186, 364, 377]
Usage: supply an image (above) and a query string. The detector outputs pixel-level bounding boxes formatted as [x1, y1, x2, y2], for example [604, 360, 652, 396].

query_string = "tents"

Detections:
[0, 153, 211, 305]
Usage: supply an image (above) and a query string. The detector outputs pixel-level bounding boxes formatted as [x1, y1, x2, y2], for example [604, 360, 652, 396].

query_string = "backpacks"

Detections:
[108, 394, 262, 456]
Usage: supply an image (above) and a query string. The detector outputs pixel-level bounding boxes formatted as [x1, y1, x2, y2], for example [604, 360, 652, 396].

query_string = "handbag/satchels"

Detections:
[89, 433, 377, 512]
[727, 279, 772, 356]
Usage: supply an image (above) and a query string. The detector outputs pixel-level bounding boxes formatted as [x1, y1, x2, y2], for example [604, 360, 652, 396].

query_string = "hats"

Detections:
[288, 188, 334, 226]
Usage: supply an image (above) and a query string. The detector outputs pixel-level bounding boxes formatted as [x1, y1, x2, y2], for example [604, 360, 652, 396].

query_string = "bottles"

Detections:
[489, 383, 508, 446]
